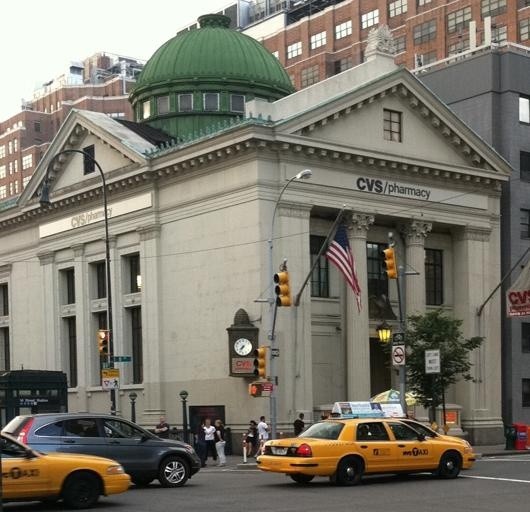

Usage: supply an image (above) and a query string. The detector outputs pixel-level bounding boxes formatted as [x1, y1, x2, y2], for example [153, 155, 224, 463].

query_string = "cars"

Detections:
[255, 401, 476, 486]
[380, 402, 474, 454]
[1, 432, 131, 510]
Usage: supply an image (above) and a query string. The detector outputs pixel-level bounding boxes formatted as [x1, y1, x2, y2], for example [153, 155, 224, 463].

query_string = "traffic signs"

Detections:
[110, 356, 132, 362]
[102, 362, 113, 368]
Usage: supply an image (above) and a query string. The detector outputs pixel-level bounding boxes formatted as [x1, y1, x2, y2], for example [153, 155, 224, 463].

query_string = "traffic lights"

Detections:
[253, 347, 266, 380]
[382, 248, 397, 280]
[273, 269, 291, 307]
[249, 383, 263, 398]
[98, 329, 108, 355]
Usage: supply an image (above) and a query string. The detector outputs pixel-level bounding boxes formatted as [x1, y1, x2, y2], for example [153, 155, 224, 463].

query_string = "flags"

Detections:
[321, 212, 363, 315]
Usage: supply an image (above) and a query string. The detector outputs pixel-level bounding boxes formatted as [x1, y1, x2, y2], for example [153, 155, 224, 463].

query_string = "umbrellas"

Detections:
[405, 389, 433, 413]
[369, 388, 418, 407]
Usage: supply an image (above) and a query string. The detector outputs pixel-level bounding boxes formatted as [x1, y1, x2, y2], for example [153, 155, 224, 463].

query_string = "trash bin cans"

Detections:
[513, 422, 528, 450]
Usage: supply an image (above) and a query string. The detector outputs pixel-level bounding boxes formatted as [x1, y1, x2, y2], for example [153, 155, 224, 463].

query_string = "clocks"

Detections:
[233, 336, 253, 356]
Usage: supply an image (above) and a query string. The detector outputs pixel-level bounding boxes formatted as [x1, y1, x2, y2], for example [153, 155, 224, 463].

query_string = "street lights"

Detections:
[269, 170, 313, 441]
[128, 393, 137, 423]
[179, 390, 188, 444]
[39, 149, 116, 417]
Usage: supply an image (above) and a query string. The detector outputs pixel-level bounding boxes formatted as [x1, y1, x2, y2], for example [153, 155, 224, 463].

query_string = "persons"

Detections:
[190, 415, 272, 468]
[372, 403, 380, 412]
[401, 410, 420, 439]
[293, 412, 306, 437]
[154, 415, 170, 439]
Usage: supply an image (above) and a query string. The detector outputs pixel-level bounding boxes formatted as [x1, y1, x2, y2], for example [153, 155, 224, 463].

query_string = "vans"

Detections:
[0, 412, 201, 488]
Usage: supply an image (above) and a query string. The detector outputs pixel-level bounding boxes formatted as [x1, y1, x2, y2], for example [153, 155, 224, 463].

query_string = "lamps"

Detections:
[376, 319, 393, 346]
